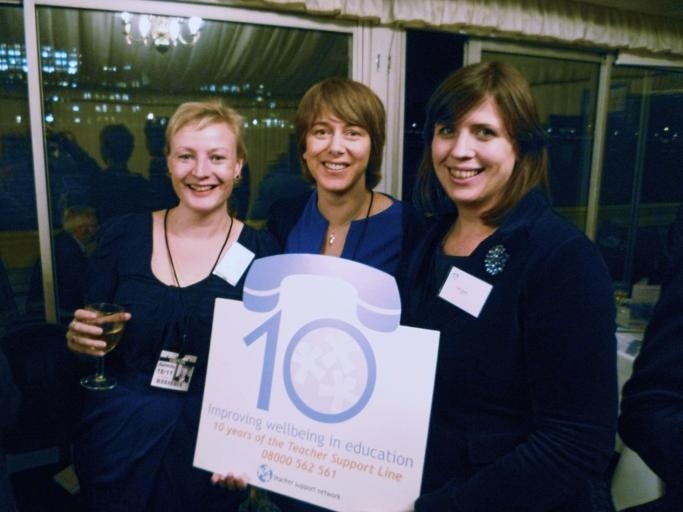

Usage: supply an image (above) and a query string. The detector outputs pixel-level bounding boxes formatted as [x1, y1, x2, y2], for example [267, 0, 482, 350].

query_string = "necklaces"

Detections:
[315, 192, 372, 246]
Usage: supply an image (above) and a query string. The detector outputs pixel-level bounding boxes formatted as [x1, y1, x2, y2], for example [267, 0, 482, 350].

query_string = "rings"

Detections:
[72, 334, 77, 344]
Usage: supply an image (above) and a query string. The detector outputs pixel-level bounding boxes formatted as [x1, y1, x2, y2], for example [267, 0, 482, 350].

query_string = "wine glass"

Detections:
[78, 302, 124, 391]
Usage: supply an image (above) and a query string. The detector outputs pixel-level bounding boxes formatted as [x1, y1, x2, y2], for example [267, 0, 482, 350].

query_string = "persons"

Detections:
[402, 62, 619, 511]
[610, 203, 682, 512]
[268, 77, 433, 511]
[31, 206, 97, 291]
[66, 102, 282, 510]
